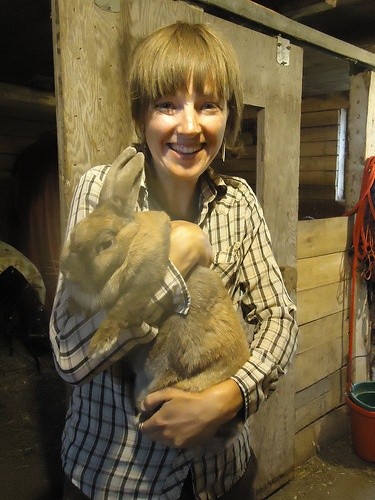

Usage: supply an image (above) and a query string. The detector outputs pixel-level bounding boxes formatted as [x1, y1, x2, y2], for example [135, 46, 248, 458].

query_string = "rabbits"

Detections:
[44, 144, 250, 463]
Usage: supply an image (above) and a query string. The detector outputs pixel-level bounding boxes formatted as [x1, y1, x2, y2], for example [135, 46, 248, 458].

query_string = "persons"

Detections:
[49, 21, 301, 500]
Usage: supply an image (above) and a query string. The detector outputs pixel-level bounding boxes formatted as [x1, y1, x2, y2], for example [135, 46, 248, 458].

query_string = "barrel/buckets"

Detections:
[345, 381, 375, 463]
